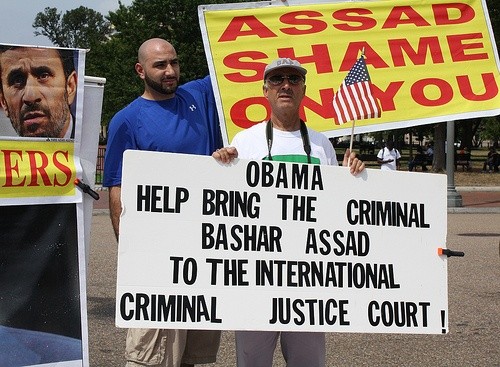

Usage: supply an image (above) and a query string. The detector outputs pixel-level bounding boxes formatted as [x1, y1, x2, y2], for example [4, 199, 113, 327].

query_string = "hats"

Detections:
[263, 57, 307, 79]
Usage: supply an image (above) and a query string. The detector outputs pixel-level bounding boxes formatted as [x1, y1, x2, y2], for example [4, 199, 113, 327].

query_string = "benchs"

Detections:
[411, 154, 470, 172]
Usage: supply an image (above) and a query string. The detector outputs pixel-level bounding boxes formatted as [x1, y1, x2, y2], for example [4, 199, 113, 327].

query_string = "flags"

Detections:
[332, 56, 381, 125]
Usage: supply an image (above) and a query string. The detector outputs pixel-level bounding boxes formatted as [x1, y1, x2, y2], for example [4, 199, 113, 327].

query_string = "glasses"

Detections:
[265, 74, 305, 86]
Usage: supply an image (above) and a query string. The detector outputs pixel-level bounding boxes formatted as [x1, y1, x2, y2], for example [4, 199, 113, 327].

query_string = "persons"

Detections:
[422, 145, 433, 171]
[481, 147, 498, 172]
[376, 141, 401, 171]
[212, 58, 365, 367]
[102, 38, 223, 367]
[0, 46, 77, 139]
